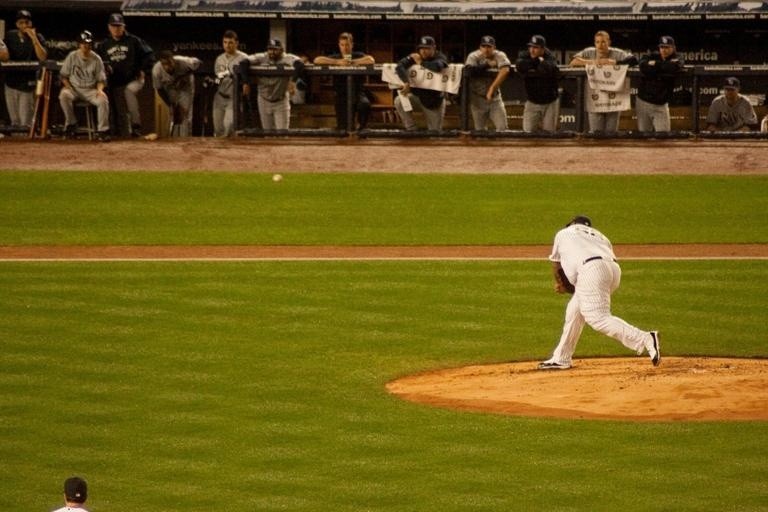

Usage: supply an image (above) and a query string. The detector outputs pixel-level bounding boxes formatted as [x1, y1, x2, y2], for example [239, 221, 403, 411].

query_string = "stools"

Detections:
[74, 98, 97, 144]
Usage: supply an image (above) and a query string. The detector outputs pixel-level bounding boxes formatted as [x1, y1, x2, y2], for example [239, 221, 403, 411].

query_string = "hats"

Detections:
[266, 37, 283, 49]
[63, 476, 90, 503]
[724, 78, 741, 89]
[109, 13, 126, 26]
[566, 216, 592, 226]
[16, 10, 32, 21]
[480, 35, 497, 47]
[528, 35, 544, 48]
[658, 35, 675, 49]
[418, 36, 437, 49]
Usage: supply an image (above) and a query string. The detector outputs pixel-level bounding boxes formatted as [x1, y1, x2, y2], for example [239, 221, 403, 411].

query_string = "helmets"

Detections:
[78, 29, 94, 44]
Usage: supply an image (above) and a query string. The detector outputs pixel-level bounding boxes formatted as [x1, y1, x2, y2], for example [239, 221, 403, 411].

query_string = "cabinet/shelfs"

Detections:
[303, 18, 468, 133]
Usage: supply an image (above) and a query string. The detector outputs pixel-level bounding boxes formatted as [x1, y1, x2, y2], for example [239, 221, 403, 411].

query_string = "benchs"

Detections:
[399, 99, 767, 136]
[284, 104, 343, 134]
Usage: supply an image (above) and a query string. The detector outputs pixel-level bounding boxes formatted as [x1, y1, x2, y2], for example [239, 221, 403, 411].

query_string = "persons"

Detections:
[2, 10, 47, 137]
[0, 40, 11, 135]
[240, 38, 304, 129]
[51, 476, 90, 512]
[313, 32, 375, 130]
[212, 30, 249, 138]
[636, 36, 681, 132]
[705, 77, 759, 132]
[96, 13, 149, 136]
[393, 36, 448, 132]
[152, 50, 203, 137]
[58, 30, 111, 142]
[516, 35, 560, 131]
[538, 217, 661, 369]
[465, 36, 511, 129]
[570, 31, 639, 132]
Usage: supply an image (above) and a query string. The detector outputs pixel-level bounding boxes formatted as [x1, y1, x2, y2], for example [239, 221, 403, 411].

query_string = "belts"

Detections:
[585, 255, 618, 264]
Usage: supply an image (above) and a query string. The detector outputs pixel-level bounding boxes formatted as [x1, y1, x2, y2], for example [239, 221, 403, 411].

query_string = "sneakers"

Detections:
[644, 331, 660, 365]
[1, 123, 159, 141]
[538, 359, 572, 371]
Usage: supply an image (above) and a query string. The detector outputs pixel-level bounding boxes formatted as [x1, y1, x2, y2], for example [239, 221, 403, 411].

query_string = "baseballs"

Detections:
[272, 174, 283, 183]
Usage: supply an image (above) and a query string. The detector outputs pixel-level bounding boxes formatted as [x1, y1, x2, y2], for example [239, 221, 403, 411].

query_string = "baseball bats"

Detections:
[28, 66, 53, 139]
